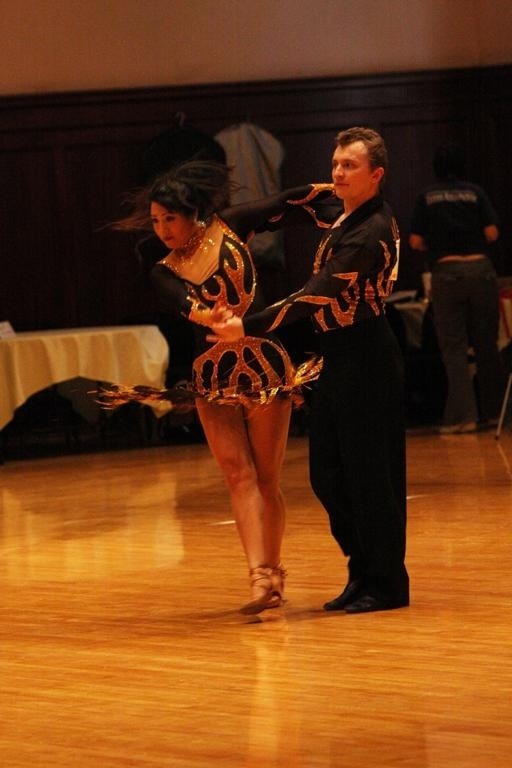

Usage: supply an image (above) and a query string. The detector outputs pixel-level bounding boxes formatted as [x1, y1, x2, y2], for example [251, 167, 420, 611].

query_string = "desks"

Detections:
[2, 322, 170, 459]
[393, 277, 511, 351]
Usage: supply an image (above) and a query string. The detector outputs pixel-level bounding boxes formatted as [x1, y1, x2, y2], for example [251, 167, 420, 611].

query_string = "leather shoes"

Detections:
[324, 582, 410, 612]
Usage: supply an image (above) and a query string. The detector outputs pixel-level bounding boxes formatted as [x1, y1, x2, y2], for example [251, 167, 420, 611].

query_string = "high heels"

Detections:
[238, 563, 288, 614]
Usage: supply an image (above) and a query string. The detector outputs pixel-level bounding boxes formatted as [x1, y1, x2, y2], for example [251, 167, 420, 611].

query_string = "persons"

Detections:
[406, 154, 503, 433]
[103, 159, 338, 620]
[204, 124, 411, 614]
